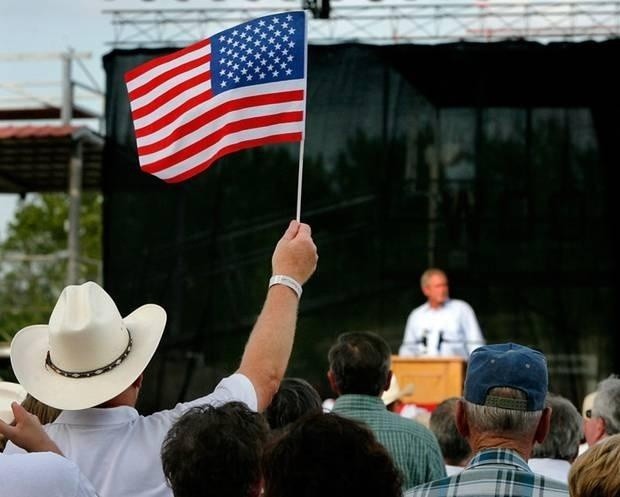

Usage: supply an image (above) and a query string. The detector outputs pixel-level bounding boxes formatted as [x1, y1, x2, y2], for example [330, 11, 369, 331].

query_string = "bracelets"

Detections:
[265, 274, 304, 298]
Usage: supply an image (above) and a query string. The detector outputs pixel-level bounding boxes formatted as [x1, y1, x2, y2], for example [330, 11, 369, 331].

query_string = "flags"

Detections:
[120, 10, 307, 186]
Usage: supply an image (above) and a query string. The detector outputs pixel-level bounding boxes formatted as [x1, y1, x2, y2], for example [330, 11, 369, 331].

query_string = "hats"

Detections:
[380, 374, 415, 408]
[463, 343, 549, 412]
[10, 281, 167, 411]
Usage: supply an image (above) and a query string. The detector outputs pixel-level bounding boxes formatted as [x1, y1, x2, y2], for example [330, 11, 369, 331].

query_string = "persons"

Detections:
[528, 375, 620, 497]
[326, 330, 448, 492]
[10, 220, 318, 496]
[0, 392, 98, 497]
[403, 342, 571, 497]
[398, 266, 488, 361]
[429, 396, 473, 475]
[160, 378, 404, 497]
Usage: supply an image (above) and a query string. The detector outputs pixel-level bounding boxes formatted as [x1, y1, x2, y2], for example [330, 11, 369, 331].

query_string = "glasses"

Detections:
[586, 410, 613, 436]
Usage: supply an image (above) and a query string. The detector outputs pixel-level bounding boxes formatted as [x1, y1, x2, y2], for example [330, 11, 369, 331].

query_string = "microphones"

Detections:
[436, 329, 444, 350]
[423, 328, 431, 353]
